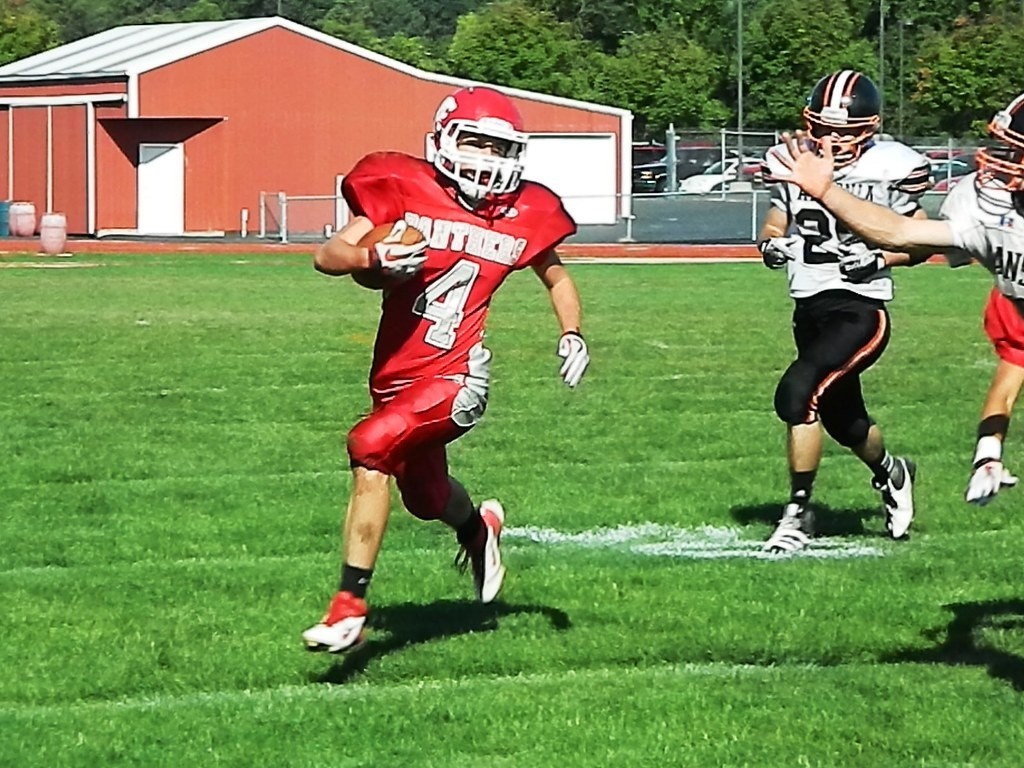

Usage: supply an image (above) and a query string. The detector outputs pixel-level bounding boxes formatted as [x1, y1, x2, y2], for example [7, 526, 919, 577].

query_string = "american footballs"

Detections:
[349, 221, 429, 290]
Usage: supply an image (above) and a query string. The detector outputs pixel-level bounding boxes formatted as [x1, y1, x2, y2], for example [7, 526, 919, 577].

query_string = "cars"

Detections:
[919, 149, 981, 191]
[627, 144, 768, 195]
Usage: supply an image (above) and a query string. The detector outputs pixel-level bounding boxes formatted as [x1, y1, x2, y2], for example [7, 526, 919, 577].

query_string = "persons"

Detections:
[753, 66, 938, 562]
[301, 85, 593, 655]
[766, 79, 1024, 502]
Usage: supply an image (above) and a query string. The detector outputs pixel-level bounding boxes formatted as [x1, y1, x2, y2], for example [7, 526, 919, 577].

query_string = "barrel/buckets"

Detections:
[15, 202, 36, 236]
[9, 203, 17, 235]
[0, 202, 9, 238]
[39, 212, 66, 254]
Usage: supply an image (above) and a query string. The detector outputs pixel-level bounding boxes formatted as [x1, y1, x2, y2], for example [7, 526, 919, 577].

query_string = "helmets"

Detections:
[975, 94, 1024, 192]
[802, 70, 881, 169]
[434, 87, 529, 200]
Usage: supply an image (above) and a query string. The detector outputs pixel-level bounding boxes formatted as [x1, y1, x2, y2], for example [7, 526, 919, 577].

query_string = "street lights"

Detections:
[899, 15, 914, 143]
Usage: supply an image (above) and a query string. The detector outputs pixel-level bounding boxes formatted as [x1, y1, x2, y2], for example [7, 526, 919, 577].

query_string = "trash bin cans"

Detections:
[8, 201, 35, 236]
[39, 212, 67, 254]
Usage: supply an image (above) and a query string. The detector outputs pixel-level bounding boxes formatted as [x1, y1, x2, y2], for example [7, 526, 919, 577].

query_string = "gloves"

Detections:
[837, 242, 885, 283]
[966, 436, 1019, 502]
[557, 331, 589, 386]
[366, 219, 427, 284]
[757, 237, 796, 269]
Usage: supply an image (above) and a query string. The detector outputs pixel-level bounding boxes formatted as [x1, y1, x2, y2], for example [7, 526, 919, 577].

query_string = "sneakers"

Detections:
[455, 498, 506, 605]
[760, 503, 813, 556]
[871, 457, 917, 540]
[301, 591, 366, 653]
